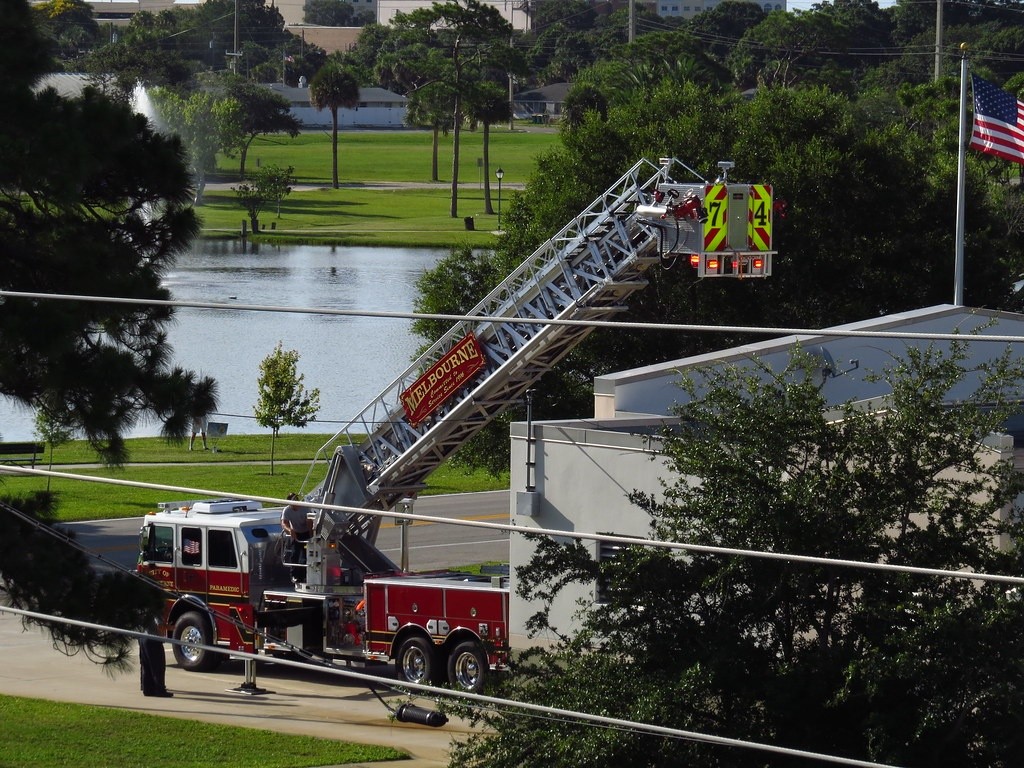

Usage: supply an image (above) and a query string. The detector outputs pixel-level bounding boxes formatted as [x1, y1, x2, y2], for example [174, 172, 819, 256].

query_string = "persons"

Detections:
[136, 576, 173, 697]
[281, 493, 310, 582]
[189, 416, 209, 450]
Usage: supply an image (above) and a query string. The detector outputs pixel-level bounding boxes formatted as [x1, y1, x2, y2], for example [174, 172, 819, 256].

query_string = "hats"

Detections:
[286, 492, 299, 501]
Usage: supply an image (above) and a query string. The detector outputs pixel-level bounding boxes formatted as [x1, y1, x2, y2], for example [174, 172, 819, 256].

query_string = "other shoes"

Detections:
[292, 577, 299, 584]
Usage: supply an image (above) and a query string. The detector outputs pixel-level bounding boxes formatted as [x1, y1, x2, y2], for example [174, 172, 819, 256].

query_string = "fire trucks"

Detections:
[130, 148, 1023, 705]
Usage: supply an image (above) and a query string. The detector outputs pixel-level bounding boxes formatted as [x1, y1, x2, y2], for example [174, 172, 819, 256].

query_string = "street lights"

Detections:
[494, 163, 505, 230]
